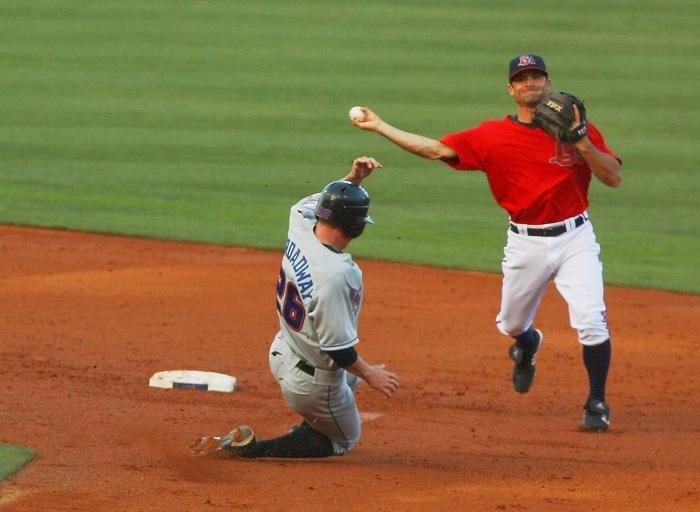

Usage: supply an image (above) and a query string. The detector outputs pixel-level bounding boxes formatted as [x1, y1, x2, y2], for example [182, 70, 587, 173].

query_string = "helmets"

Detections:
[316, 181, 374, 239]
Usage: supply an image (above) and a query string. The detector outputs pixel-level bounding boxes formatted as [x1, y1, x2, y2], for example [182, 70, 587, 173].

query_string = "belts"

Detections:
[296, 360, 315, 376]
[510, 217, 584, 236]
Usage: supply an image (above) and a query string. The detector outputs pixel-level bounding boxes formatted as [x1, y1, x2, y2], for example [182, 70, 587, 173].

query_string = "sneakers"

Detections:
[585, 396, 609, 430]
[190, 425, 258, 458]
[514, 328, 543, 392]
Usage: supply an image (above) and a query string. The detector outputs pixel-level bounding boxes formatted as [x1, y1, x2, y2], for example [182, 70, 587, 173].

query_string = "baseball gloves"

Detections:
[532, 91, 588, 146]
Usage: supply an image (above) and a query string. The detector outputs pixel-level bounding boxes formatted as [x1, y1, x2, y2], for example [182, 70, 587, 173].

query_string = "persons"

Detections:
[181, 157, 399, 458]
[352, 55, 622, 431]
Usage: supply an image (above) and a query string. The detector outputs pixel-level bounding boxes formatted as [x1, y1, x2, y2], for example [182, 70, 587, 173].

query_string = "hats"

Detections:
[509, 54, 548, 83]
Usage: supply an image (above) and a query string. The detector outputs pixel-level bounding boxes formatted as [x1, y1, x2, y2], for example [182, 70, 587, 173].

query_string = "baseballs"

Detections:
[349, 106, 365, 123]
